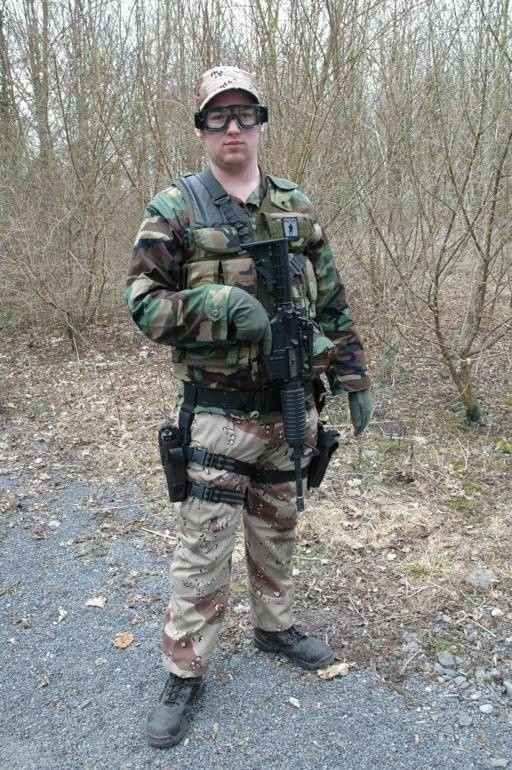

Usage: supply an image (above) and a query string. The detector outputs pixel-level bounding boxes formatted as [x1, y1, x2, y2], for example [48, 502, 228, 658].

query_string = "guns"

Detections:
[233, 235, 320, 511]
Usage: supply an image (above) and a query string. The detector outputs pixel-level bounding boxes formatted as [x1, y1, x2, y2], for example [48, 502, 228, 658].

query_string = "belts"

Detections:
[193, 378, 314, 412]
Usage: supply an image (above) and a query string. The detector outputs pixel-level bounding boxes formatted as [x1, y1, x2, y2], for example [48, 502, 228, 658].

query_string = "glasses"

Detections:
[193, 103, 269, 133]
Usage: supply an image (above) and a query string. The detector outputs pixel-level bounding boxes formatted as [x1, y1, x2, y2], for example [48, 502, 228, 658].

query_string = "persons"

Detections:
[124, 68, 374, 748]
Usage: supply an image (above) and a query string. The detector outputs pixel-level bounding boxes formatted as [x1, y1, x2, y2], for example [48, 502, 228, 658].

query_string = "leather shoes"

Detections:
[253, 625, 335, 671]
[145, 671, 205, 748]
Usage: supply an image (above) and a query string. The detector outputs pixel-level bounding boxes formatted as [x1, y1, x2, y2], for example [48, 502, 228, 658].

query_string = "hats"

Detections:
[193, 66, 261, 111]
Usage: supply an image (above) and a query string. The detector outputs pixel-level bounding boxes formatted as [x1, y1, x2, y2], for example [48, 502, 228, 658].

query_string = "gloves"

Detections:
[229, 286, 273, 356]
[348, 387, 375, 436]
